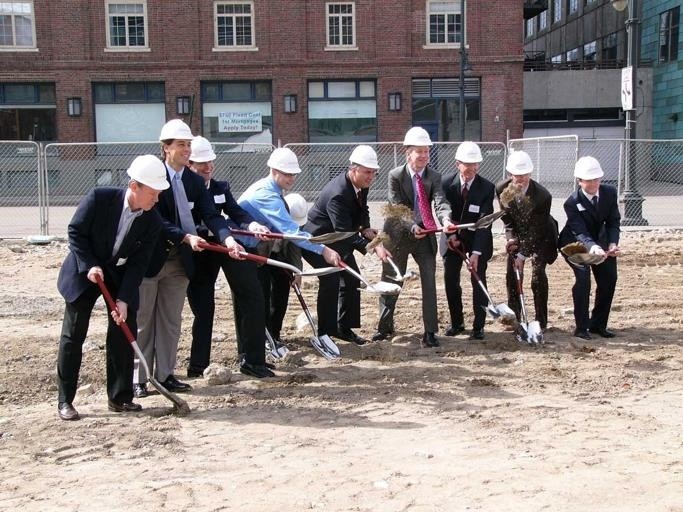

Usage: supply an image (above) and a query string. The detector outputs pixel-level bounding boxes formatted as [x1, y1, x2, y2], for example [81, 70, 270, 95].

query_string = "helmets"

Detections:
[505, 150, 533, 175]
[267, 148, 302, 174]
[189, 135, 216, 162]
[160, 118, 194, 140]
[284, 193, 308, 227]
[127, 154, 170, 191]
[403, 126, 433, 146]
[348, 145, 381, 169]
[455, 142, 483, 163]
[574, 156, 603, 181]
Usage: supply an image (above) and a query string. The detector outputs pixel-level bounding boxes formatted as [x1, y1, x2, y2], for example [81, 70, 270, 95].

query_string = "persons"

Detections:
[372, 126, 456, 347]
[133, 119, 247, 400]
[266, 193, 309, 353]
[557, 157, 617, 341]
[186, 136, 274, 379]
[54, 154, 171, 420]
[496, 150, 559, 333]
[300, 144, 392, 345]
[220, 147, 341, 379]
[439, 140, 496, 340]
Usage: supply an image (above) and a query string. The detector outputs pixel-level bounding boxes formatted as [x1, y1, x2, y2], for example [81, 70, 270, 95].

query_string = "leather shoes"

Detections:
[240, 358, 275, 378]
[590, 326, 616, 338]
[57, 402, 78, 420]
[266, 362, 276, 369]
[265, 340, 282, 350]
[575, 328, 591, 339]
[187, 367, 204, 377]
[108, 398, 142, 412]
[161, 374, 190, 392]
[133, 383, 148, 397]
[473, 325, 484, 338]
[372, 326, 394, 341]
[446, 323, 464, 336]
[423, 332, 439, 346]
[334, 327, 368, 344]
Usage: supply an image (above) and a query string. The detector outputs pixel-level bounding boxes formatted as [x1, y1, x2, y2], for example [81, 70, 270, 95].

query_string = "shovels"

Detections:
[449, 239, 516, 325]
[385, 256, 419, 282]
[567, 250, 615, 268]
[284, 269, 341, 360]
[416, 210, 506, 235]
[338, 261, 402, 296]
[197, 241, 347, 277]
[265, 325, 291, 365]
[229, 227, 356, 245]
[506, 248, 546, 345]
[93, 271, 183, 408]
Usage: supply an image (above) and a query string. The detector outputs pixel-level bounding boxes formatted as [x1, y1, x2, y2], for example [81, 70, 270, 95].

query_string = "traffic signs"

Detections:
[620, 65, 633, 111]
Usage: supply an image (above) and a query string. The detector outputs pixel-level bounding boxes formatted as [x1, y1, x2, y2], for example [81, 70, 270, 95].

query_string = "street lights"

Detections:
[611, 0, 650, 227]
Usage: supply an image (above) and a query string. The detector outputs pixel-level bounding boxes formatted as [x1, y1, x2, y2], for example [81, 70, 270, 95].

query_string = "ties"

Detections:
[358, 191, 362, 207]
[174, 173, 198, 235]
[462, 182, 469, 199]
[415, 173, 437, 230]
[112, 213, 135, 256]
[592, 196, 598, 208]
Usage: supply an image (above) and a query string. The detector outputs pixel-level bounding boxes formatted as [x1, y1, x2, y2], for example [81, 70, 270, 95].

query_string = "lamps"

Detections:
[283, 93, 298, 113]
[387, 92, 402, 111]
[175, 95, 192, 116]
[66, 96, 83, 117]
